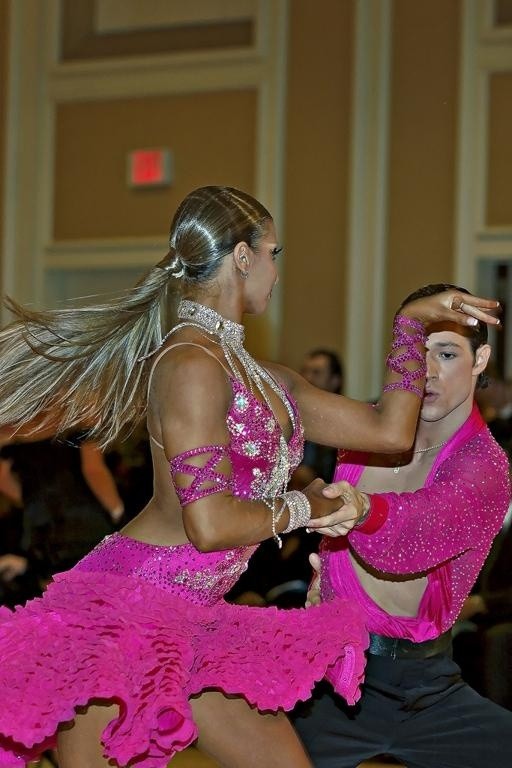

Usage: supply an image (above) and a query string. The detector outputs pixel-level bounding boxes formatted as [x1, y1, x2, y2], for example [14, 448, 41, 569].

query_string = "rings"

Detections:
[456, 299, 463, 308]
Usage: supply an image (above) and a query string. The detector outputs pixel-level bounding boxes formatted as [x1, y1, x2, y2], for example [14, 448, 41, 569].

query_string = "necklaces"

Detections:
[391, 441, 447, 472]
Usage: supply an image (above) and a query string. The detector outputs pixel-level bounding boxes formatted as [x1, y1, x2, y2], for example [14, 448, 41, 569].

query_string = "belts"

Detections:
[364, 626, 455, 662]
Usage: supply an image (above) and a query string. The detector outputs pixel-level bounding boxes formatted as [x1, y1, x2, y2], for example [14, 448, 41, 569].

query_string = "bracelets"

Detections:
[275, 491, 316, 532]
[110, 502, 124, 522]
[378, 314, 429, 398]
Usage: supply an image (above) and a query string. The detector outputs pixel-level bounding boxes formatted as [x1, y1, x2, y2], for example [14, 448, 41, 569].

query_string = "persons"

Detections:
[250, 345, 343, 598]
[284, 282, 512, 766]
[0, 183, 502, 768]
[0, 386, 127, 597]
